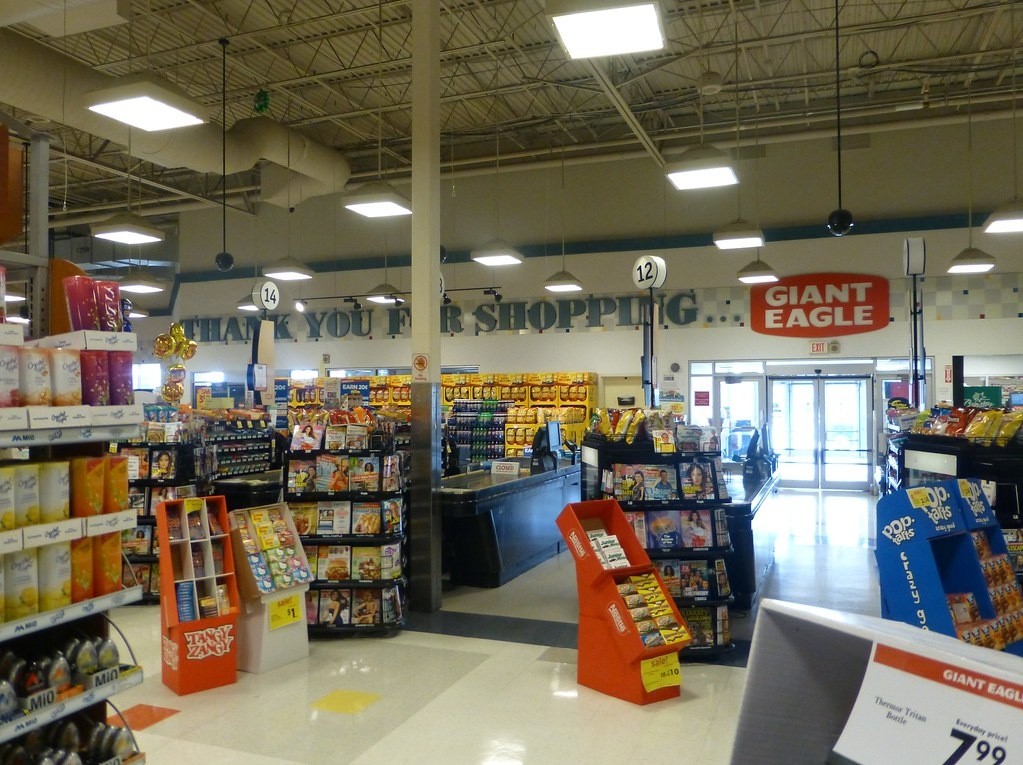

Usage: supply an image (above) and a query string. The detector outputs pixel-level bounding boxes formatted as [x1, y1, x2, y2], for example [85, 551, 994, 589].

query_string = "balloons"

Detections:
[154, 323, 198, 402]
[122, 298, 134, 332]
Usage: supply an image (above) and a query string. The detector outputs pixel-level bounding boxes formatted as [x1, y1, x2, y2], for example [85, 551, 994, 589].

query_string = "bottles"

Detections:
[447, 398, 515, 464]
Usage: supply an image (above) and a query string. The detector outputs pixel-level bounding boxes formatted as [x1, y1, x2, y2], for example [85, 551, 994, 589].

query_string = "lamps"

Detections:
[4, 1, 1023, 331]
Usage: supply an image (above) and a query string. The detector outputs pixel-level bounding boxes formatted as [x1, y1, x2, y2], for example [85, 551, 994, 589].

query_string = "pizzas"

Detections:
[292, 515, 312, 534]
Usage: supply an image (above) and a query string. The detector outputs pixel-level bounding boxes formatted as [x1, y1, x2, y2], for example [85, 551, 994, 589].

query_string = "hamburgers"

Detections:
[326, 560, 349, 579]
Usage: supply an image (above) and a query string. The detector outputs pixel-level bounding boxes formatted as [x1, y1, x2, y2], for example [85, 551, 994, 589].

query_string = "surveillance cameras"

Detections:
[828, 209, 854, 236]
[215, 252, 234, 270]
[440, 244, 447, 265]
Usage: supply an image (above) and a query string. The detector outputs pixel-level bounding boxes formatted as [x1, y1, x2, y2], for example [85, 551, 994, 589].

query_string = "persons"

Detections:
[709, 430, 718, 451]
[296, 425, 320, 450]
[324, 591, 349, 624]
[686, 464, 707, 500]
[621, 465, 713, 500]
[386, 501, 400, 525]
[660, 433, 675, 451]
[329, 460, 350, 490]
[365, 463, 378, 490]
[355, 460, 365, 488]
[353, 592, 378, 623]
[654, 470, 675, 499]
[657, 563, 728, 595]
[132, 423, 183, 590]
[686, 511, 710, 546]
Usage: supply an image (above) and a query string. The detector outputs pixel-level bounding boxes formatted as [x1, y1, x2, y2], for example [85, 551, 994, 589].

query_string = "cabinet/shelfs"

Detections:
[0, 400, 150, 765]
[287, 372, 598, 638]
[174, 430, 288, 496]
[883, 428, 1023, 581]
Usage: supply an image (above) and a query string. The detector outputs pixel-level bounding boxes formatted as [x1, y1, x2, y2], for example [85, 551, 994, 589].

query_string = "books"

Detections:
[613, 464, 645, 501]
[166, 422, 184, 443]
[679, 560, 710, 601]
[290, 425, 403, 624]
[624, 509, 731, 548]
[716, 559, 730, 596]
[655, 559, 681, 598]
[121, 422, 177, 594]
[652, 426, 719, 452]
[288, 459, 316, 491]
[383, 456, 401, 491]
[681, 606, 730, 647]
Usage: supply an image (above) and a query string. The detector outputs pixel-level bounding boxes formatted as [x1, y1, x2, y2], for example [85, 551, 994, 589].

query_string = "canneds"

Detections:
[0, 343, 133, 408]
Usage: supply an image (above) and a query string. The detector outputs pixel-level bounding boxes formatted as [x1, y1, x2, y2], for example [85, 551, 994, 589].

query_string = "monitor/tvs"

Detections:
[745, 423, 770, 460]
[532, 426, 547, 449]
[546, 421, 563, 451]
[1009, 392, 1023, 406]
